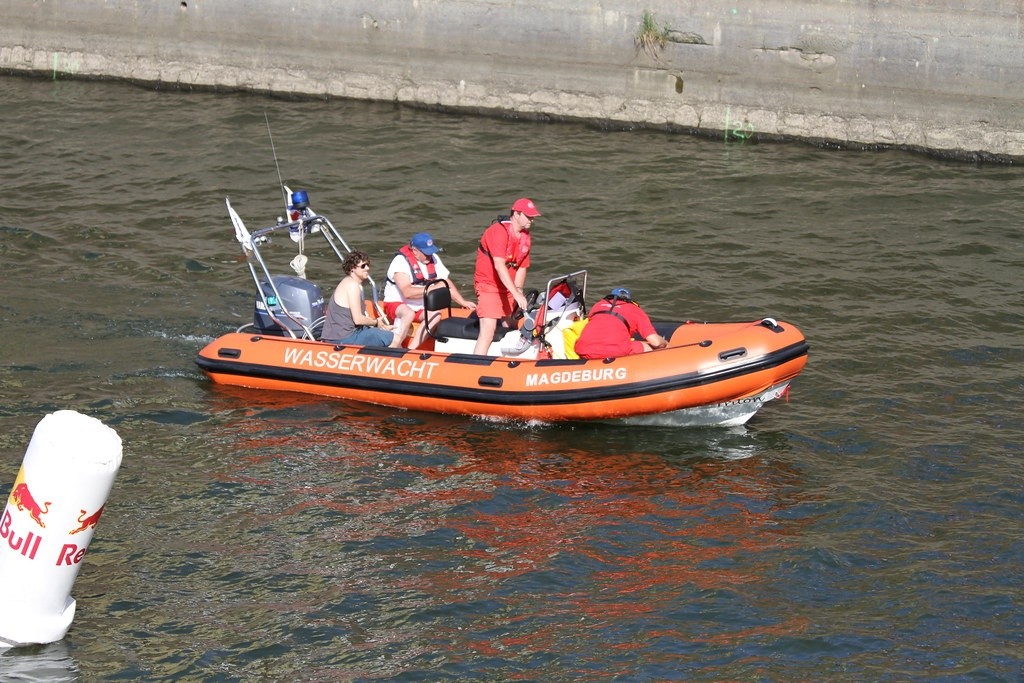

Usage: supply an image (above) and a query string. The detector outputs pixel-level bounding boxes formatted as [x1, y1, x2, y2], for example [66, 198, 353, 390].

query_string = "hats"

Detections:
[413, 233, 438, 255]
[511, 198, 541, 217]
[612, 287, 631, 298]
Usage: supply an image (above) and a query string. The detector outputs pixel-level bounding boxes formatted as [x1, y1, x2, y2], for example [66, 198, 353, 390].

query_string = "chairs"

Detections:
[424, 279, 515, 342]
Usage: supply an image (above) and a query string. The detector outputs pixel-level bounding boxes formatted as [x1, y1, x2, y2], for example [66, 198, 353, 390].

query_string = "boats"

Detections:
[194, 189, 809, 429]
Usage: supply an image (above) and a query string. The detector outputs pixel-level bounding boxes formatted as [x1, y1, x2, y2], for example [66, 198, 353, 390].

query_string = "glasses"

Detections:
[354, 263, 369, 269]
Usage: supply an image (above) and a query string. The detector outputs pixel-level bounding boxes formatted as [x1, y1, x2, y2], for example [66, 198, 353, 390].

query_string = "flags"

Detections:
[287, 190, 322, 242]
[226, 205, 253, 250]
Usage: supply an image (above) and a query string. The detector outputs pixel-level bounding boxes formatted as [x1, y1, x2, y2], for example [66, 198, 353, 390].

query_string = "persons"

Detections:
[575, 287, 667, 359]
[384, 232, 477, 349]
[320, 250, 394, 347]
[473, 198, 542, 356]
